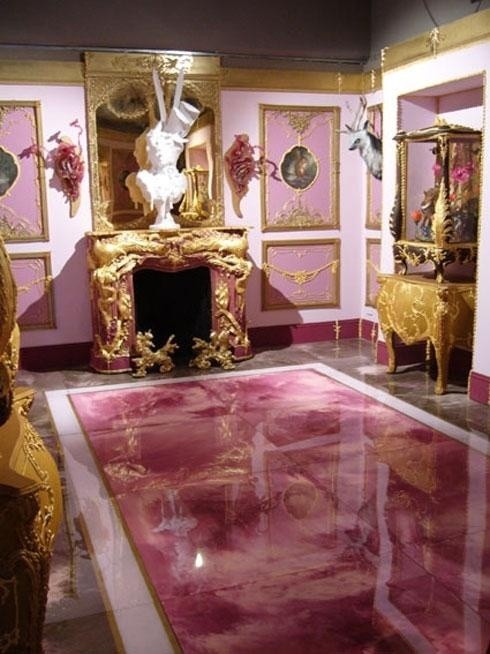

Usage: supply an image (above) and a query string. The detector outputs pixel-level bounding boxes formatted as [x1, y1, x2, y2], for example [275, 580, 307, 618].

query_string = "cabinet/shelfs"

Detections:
[387, 117, 481, 283]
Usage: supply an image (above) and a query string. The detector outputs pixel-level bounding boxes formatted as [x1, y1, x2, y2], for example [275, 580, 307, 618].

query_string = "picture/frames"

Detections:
[7, 251, 57, 332]
[361, 441, 379, 503]
[363, 396, 382, 443]
[263, 402, 341, 447]
[0, 99, 50, 244]
[261, 238, 340, 312]
[364, 103, 384, 231]
[365, 238, 380, 309]
[262, 442, 340, 564]
[257, 103, 341, 233]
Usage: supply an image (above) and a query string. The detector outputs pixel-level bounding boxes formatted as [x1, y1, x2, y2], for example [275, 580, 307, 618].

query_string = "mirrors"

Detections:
[83, 51, 226, 232]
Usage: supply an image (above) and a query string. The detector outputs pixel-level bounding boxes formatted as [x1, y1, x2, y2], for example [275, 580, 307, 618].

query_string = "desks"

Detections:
[371, 421, 468, 497]
[376, 272, 477, 396]
